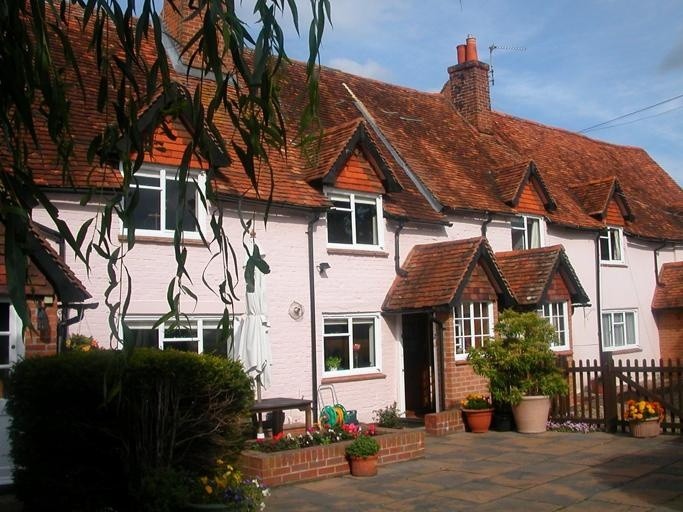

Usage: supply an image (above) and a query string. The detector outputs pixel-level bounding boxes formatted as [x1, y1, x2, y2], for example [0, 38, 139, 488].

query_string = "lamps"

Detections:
[316, 262, 330, 273]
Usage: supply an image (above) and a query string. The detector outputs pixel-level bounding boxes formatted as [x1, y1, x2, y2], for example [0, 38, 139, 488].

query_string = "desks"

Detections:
[249, 396, 314, 433]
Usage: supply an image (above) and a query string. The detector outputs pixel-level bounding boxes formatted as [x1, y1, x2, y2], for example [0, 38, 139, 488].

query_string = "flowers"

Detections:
[459, 392, 491, 409]
[624, 398, 664, 419]
[63, 329, 99, 351]
[177, 456, 272, 512]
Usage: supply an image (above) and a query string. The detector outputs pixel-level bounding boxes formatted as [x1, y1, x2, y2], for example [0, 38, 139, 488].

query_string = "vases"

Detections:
[184, 499, 235, 512]
[628, 416, 661, 439]
[461, 407, 497, 433]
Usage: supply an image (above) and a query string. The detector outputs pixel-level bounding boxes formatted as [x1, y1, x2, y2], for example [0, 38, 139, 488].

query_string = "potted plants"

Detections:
[344, 435, 380, 478]
[591, 377, 604, 394]
[326, 354, 343, 372]
[463, 307, 569, 434]
